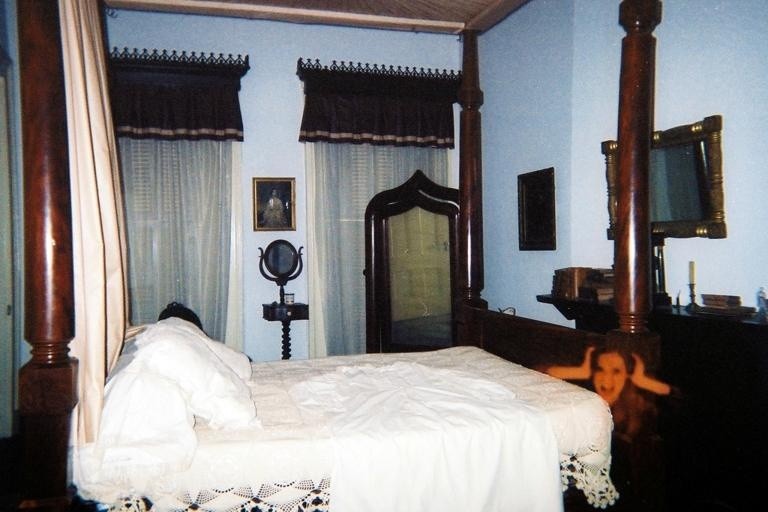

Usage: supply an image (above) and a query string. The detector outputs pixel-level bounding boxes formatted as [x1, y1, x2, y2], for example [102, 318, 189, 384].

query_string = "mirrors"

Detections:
[362, 167, 466, 353]
[600, 114, 728, 240]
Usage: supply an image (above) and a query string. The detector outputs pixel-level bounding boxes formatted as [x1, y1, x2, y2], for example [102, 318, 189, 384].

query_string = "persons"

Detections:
[530, 332, 686, 446]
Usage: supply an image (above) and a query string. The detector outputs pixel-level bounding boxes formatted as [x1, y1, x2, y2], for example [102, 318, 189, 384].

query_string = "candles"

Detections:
[688, 260, 695, 285]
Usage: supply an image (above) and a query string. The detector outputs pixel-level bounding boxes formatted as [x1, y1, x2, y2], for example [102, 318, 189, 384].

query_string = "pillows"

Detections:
[98, 316, 259, 446]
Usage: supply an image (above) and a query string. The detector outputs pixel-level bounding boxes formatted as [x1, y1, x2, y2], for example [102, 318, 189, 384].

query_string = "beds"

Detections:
[13, 305, 642, 512]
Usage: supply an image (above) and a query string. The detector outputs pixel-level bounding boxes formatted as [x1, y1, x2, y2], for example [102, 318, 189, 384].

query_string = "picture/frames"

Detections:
[518, 167, 557, 250]
[253, 178, 297, 232]
